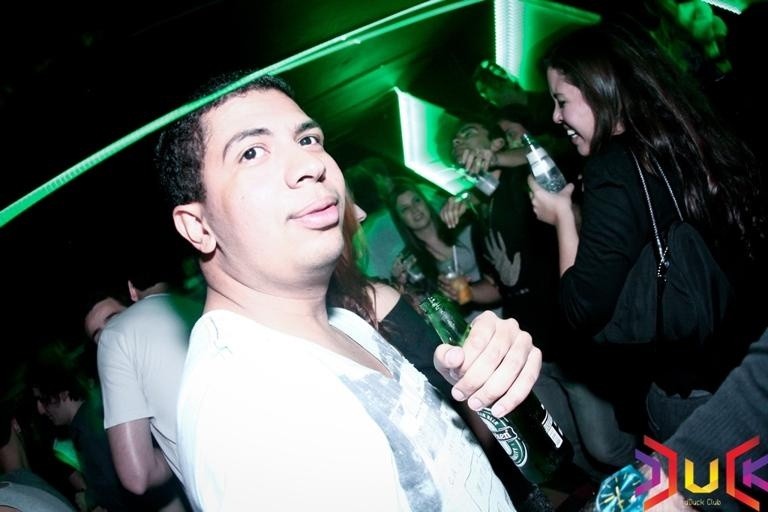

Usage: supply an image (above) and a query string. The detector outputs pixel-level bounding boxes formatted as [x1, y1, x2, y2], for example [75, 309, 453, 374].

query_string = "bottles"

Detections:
[516, 132, 568, 194]
[418, 292, 576, 488]
[451, 157, 501, 196]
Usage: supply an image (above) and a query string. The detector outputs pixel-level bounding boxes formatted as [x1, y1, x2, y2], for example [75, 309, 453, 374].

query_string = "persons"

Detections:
[0, 23, 768, 511]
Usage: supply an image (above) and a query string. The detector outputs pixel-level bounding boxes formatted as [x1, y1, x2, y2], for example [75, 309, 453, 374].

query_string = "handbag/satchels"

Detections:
[591, 221, 729, 345]
